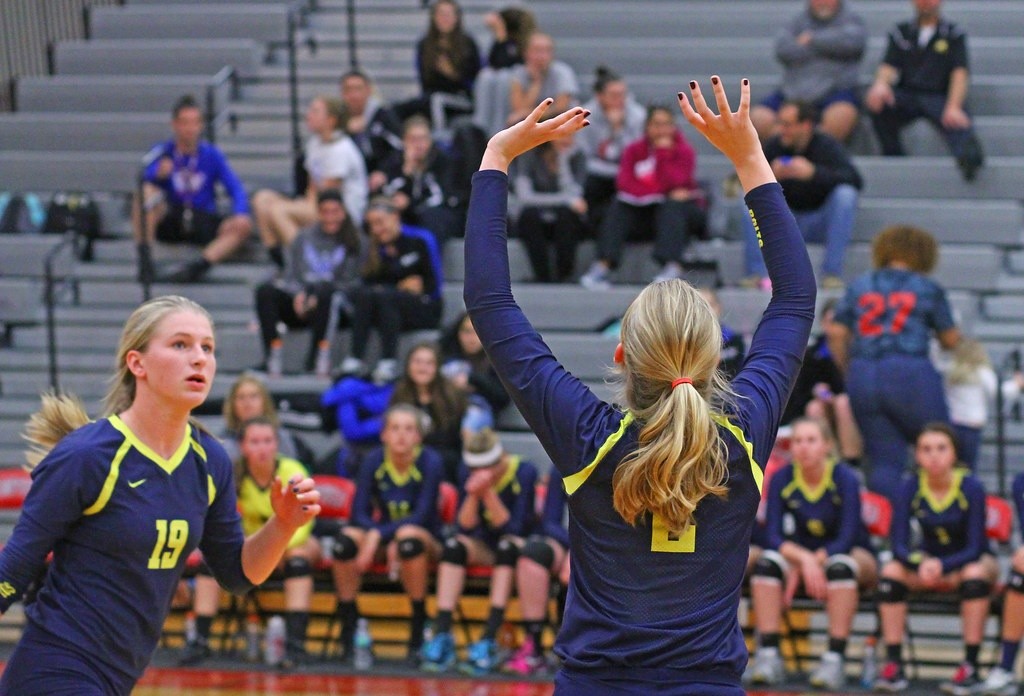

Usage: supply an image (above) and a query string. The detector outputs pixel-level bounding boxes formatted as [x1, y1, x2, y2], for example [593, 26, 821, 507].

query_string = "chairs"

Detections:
[0, 463, 1013, 683]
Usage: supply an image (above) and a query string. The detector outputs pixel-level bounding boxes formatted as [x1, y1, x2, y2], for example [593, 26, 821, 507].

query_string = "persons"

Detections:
[460, 75, 819, 696]
[1, 0, 1024, 696]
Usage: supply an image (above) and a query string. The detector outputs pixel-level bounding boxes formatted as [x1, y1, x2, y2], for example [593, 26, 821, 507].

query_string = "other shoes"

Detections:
[979, 668, 1018, 696]
[281, 644, 306, 669]
[739, 276, 762, 290]
[871, 662, 909, 692]
[820, 276, 845, 289]
[420, 633, 456, 674]
[655, 267, 679, 284]
[750, 647, 785, 685]
[371, 359, 396, 379]
[809, 652, 846, 691]
[332, 356, 365, 379]
[500, 639, 547, 678]
[940, 662, 982, 696]
[178, 642, 212, 667]
[580, 266, 608, 287]
[457, 639, 499, 678]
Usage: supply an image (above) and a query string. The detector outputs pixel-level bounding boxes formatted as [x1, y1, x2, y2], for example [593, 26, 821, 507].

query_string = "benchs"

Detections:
[0, 0, 1024, 509]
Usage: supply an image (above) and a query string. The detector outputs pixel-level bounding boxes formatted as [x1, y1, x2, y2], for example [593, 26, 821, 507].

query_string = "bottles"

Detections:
[353, 618, 374, 670]
[184, 611, 198, 646]
[860, 638, 879, 689]
[246, 614, 259, 661]
[265, 615, 288, 667]
[502, 623, 516, 650]
